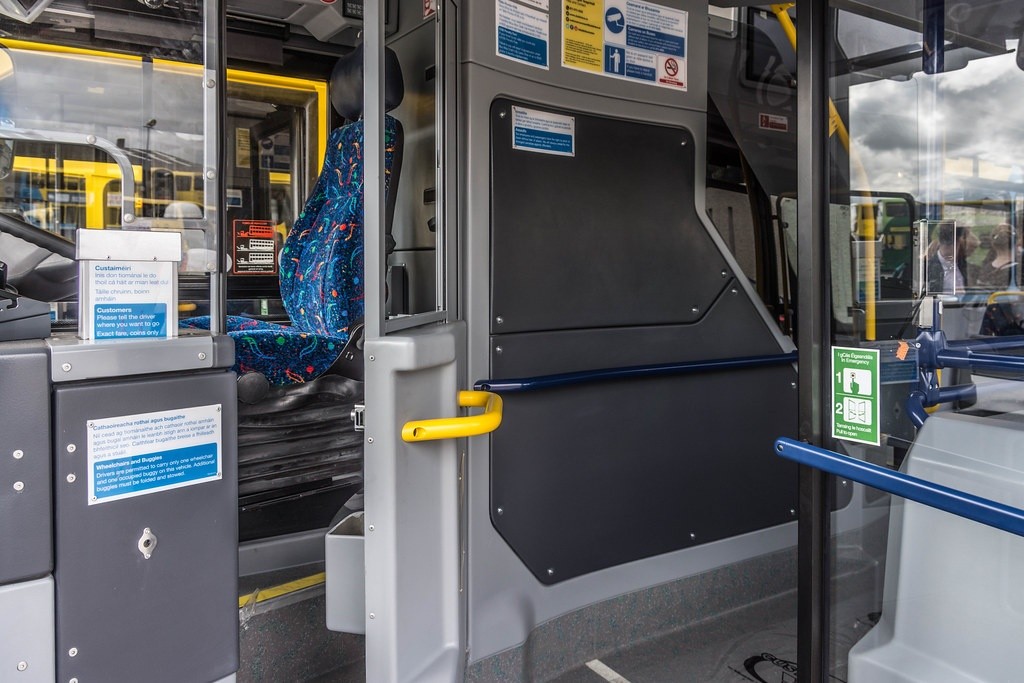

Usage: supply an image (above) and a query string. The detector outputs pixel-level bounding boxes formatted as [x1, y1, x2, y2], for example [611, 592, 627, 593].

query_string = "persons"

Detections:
[924, 219, 980, 294]
[975, 210, 1024, 295]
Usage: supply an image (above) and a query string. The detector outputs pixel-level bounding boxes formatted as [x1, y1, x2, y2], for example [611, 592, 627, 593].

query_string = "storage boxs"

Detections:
[325, 509, 365, 636]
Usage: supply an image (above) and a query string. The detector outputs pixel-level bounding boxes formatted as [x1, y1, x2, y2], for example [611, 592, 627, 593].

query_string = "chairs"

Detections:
[176, 45, 406, 406]
